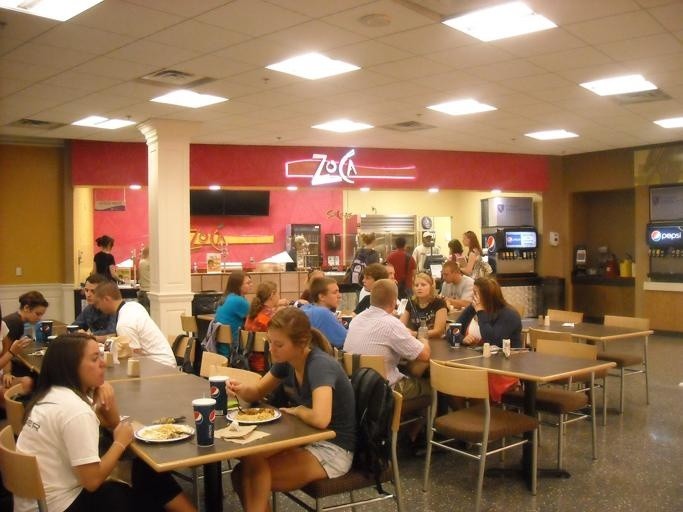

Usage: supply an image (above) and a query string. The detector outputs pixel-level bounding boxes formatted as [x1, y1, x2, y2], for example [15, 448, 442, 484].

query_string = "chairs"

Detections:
[0, 307, 655, 512]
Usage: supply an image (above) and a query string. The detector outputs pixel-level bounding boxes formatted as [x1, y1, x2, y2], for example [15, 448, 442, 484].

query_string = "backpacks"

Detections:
[172, 334, 208, 376]
[201, 320, 221, 353]
[229, 327, 255, 371]
[343, 250, 375, 288]
[351, 367, 394, 476]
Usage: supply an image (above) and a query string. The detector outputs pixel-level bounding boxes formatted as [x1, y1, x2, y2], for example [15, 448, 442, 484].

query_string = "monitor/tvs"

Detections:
[423, 254, 444, 269]
[190, 189, 225, 215]
[225, 190, 271, 216]
[496, 228, 538, 252]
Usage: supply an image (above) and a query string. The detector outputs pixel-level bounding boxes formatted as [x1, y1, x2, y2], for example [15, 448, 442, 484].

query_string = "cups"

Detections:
[341, 316, 351, 328]
[65, 325, 78, 334]
[40, 321, 52, 343]
[192, 397, 215, 447]
[209, 375, 230, 416]
[126, 360, 139, 377]
[47, 335, 58, 344]
[449, 324, 462, 349]
[103, 352, 112, 366]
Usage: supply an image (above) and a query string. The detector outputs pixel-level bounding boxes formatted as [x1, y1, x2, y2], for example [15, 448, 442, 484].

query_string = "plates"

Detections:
[228, 407, 282, 423]
[133, 423, 194, 442]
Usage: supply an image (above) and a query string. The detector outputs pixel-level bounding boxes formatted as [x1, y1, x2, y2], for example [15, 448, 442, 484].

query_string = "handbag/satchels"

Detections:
[192, 293, 224, 315]
[473, 261, 493, 280]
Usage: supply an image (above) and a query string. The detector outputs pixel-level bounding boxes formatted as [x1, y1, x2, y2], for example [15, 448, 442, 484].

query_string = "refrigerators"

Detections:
[284, 223, 321, 269]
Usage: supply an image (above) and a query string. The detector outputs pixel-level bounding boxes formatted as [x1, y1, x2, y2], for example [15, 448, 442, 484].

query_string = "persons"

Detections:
[9, 330, 200, 511]
[223, 305, 358, 511]
[69, 275, 116, 335]
[212, 270, 351, 375]
[138, 247, 151, 306]
[344, 232, 492, 338]
[93, 235, 126, 284]
[1, 321, 32, 401]
[446, 277, 521, 449]
[89, 280, 177, 368]
[2, 291, 49, 402]
[343, 278, 433, 449]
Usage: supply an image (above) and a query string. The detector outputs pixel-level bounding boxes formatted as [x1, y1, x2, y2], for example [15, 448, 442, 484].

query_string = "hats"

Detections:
[423, 232, 432, 238]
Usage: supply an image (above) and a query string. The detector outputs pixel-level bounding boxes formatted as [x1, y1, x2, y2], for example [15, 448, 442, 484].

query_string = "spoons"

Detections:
[228, 380, 245, 414]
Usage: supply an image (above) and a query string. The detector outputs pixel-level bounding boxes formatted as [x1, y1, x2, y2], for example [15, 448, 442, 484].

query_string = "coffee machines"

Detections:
[576, 247, 593, 280]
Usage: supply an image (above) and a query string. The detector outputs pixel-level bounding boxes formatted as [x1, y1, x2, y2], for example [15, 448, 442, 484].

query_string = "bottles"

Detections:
[249, 257, 254, 264]
[544, 316, 549, 326]
[23, 323, 32, 339]
[537, 315, 543, 325]
[34, 321, 40, 342]
[288, 298, 295, 306]
[417, 320, 427, 339]
[483, 343, 490, 357]
[192, 261, 196, 271]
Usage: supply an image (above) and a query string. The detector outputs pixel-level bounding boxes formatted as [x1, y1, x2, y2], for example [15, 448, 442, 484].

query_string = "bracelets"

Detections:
[113, 441, 125, 450]
[8, 349, 15, 357]
[294, 404, 305, 415]
